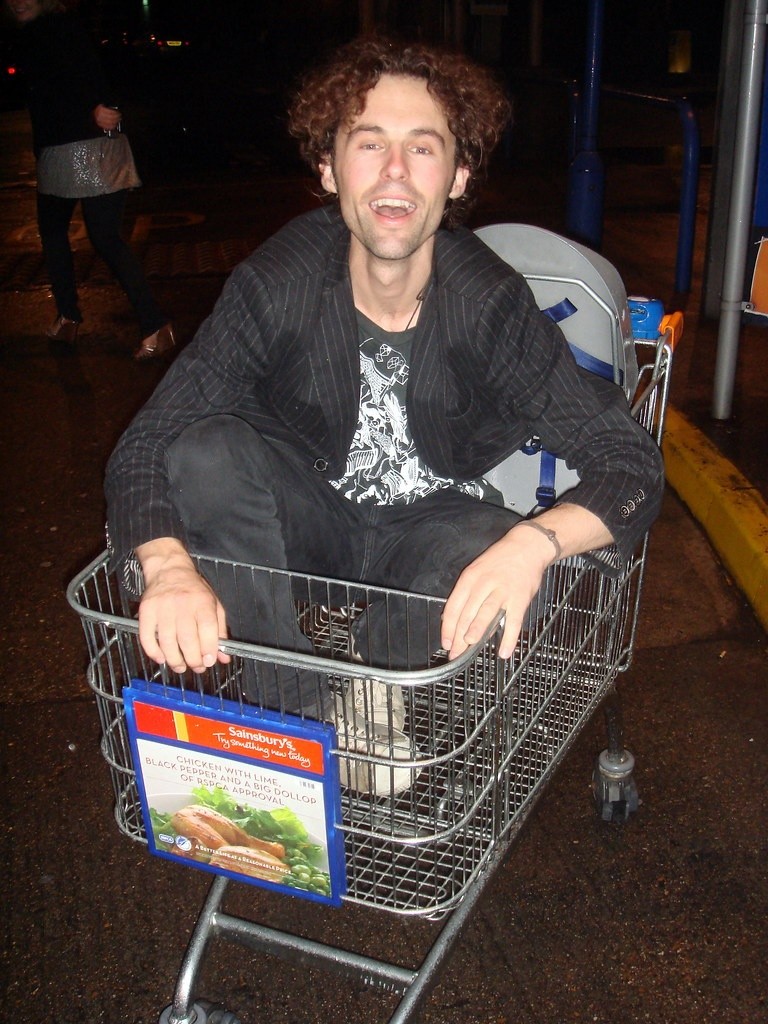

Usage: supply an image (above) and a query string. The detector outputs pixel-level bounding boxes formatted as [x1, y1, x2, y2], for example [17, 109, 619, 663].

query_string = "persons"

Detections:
[101, 32, 666, 798]
[0, 0, 177, 365]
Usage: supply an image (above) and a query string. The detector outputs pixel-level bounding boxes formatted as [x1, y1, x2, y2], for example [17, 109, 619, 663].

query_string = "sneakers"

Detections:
[320, 691, 424, 795]
[346, 634, 405, 732]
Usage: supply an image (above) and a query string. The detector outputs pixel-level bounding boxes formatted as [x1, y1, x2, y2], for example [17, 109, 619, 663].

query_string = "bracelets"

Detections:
[514, 520, 561, 565]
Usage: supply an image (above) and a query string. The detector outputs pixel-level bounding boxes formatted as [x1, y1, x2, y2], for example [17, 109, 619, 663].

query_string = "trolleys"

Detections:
[67, 269, 684, 1024]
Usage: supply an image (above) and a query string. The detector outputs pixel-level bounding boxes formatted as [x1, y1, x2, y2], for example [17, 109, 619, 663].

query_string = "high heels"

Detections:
[43, 315, 82, 344]
[131, 324, 176, 361]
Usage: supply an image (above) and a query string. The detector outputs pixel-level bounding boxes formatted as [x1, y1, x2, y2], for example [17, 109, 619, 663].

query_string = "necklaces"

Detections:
[404, 278, 431, 330]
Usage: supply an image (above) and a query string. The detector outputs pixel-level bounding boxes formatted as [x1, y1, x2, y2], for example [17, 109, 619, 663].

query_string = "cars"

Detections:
[120, 19, 192, 57]
